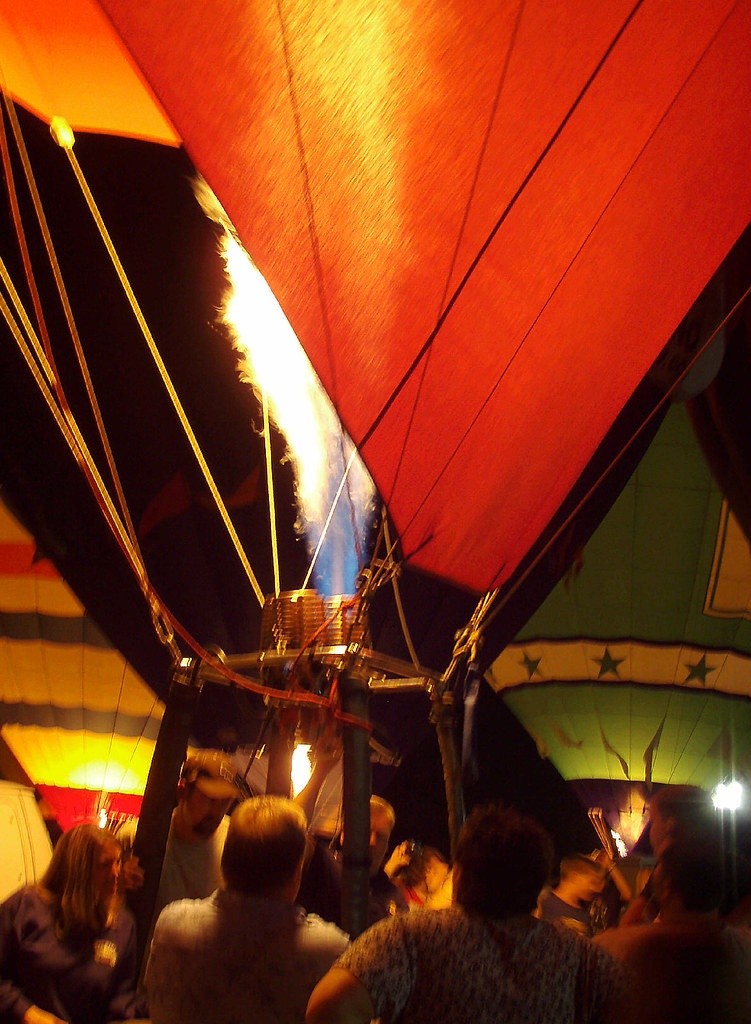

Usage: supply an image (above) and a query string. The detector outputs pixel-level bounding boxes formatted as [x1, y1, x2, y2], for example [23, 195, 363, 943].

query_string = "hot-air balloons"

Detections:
[0, 500, 168, 855]
[479, 395, 751, 900]
[0, 0, 751, 757]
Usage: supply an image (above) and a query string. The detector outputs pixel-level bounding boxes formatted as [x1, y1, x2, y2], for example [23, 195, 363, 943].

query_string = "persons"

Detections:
[0, 823, 140, 1024]
[529, 852, 606, 937]
[114, 748, 239, 994]
[587, 784, 751, 1024]
[140, 792, 354, 1024]
[291, 719, 409, 940]
[383, 838, 462, 917]
[304, 799, 651, 1024]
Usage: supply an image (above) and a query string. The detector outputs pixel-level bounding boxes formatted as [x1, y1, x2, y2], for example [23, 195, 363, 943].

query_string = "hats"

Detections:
[183, 749, 243, 800]
